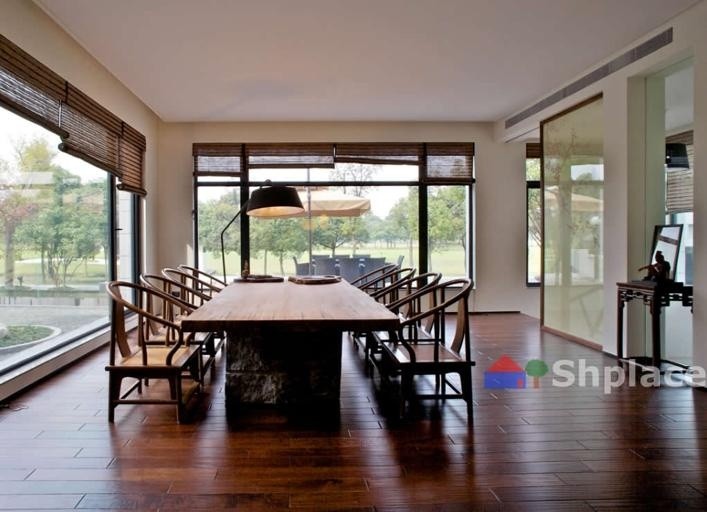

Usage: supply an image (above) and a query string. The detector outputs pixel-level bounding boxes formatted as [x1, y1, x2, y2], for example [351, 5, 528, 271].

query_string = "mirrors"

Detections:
[648, 223, 684, 281]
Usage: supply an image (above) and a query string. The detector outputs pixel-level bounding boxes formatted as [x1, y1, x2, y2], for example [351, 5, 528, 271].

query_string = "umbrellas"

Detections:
[292, 190, 372, 218]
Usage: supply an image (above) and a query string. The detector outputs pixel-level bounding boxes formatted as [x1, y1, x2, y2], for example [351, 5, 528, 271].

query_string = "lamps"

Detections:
[219, 177, 305, 286]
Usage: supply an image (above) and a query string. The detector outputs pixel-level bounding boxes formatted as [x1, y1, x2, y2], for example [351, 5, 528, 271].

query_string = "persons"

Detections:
[638, 250, 670, 282]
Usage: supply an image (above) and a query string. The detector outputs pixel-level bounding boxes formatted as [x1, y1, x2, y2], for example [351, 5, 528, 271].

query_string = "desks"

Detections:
[615, 281, 693, 389]
